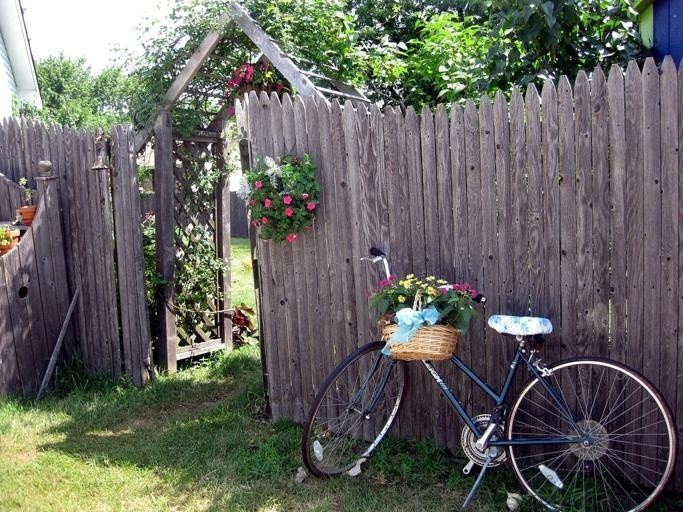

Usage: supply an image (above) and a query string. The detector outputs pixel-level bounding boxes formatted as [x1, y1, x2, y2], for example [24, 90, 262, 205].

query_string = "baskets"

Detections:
[382, 289, 461, 361]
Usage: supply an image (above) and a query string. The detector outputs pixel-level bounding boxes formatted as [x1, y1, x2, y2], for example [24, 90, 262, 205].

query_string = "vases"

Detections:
[238, 82, 271, 106]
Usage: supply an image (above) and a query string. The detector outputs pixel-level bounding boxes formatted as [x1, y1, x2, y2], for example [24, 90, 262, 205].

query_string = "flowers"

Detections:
[222, 62, 284, 119]
[368, 272, 480, 333]
[233, 151, 325, 246]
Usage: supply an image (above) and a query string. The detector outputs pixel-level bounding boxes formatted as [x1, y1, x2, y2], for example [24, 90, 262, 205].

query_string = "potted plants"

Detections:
[17, 177, 35, 225]
[0, 222, 20, 258]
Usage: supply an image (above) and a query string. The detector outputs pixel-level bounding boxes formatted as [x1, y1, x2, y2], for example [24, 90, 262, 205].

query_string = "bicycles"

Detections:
[302, 246, 679, 512]
